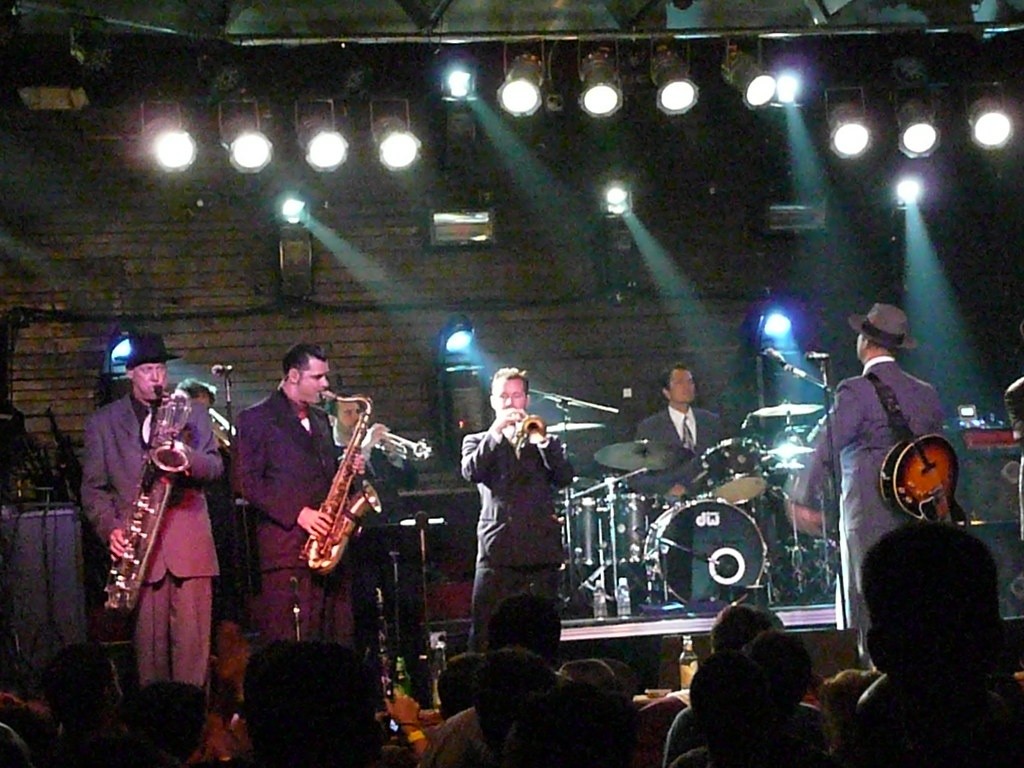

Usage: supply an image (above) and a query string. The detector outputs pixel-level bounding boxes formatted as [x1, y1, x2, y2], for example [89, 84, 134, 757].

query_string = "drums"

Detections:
[570, 491, 667, 570]
[700, 437, 768, 505]
[642, 499, 769, 613]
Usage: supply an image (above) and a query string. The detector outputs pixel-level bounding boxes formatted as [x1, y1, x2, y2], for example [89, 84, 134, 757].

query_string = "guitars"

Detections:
[879, 433, 964, 528]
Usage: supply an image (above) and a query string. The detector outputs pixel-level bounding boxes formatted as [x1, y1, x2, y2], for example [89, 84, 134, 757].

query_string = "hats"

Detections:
[848, 303, 917, 350]
[114, 332, 182, 362]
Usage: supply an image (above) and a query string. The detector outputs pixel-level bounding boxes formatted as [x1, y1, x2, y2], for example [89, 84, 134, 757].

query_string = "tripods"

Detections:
[565, 448, 651, 606]
[772, 411, 834, 600]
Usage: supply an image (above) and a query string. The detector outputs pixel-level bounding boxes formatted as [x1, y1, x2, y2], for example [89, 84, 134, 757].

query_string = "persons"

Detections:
[0, 521, 1024, 768]
[1004, 320, 1024, 544]
[637, 363, 724, 484]
[459, 368, 574, 650]
[784, 303, 946, 630]
[81, 337, 225, 686]
[230, 342, 364, 638]
[327, 391, 416, 475]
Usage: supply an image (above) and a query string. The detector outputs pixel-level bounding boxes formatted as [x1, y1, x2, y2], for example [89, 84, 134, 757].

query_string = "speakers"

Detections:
[945, 426, 1024, 618]
[0, 501, 86, 682]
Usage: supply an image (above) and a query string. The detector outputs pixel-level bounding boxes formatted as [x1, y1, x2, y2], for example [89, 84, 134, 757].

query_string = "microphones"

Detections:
[211, 365, 233, 376]
[764, 348, 791, 371]
[805, 352, 830, 363]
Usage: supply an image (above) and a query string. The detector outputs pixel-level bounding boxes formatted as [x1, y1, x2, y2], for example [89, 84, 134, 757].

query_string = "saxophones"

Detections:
[298, 391, 386, 576]
[102, 385, 193, 618]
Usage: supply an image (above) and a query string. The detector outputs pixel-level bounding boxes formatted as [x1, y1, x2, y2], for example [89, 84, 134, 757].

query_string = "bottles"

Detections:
[414, 655, 434, 710]
[392, 657, 411, 703]
[616, 577, 631, 617]
[593, 580, 608, 618]
[680, 636, 698, 690]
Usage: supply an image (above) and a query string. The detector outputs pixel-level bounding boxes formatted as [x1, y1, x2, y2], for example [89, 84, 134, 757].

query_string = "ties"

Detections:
[297, 409, 311, 435]
[683, 416, 695, 454]
[142, 409, 153, 445]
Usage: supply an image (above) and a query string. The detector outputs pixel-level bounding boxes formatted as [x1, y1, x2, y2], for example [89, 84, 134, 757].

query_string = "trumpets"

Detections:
[513, 412, 547, 444]
[365, 424, 438, 466]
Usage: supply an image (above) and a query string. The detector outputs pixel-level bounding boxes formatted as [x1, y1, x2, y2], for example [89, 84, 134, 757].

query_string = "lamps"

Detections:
[293, 98, 350, 173]
[577, 33, 623, 117]
[721, 31, 778, 109]
[369, 97, 423, 173]
[140, 100, 199, 174]
[495, 35, 547, 118]
[824, 85, 873, 160]
[963, 81, 1015, 151]
[439, 43, 479, 101]
[646, 29, 699, 116]
[891, 82, 942, 159]
[217, 98, 275, 175]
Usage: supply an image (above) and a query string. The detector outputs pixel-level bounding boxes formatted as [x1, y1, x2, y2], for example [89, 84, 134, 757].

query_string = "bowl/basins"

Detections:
[646, 690, 671, 698]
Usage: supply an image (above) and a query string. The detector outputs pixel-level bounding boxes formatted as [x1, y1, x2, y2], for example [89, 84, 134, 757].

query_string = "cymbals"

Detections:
[593, 439, 693, 473]
[547, 422, 604, 432]
[751, 404, 826, 417]
[759, 442, 817, 456]
